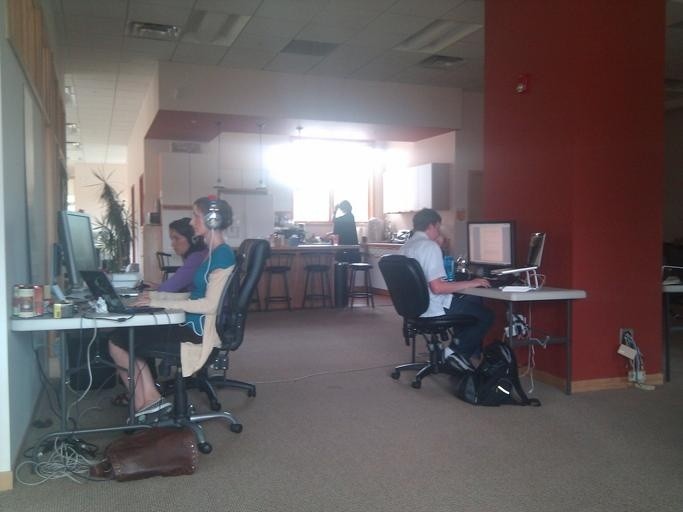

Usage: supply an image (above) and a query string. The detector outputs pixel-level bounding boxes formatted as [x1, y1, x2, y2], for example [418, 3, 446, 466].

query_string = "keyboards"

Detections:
[119, 288, 138, 296]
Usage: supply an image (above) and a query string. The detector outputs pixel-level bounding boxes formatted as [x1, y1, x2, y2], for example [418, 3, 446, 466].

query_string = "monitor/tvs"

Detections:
[467, 220, 513, 281]
[58, 211, 97, 299]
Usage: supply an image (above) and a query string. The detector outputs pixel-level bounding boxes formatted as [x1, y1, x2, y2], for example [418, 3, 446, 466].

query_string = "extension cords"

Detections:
[504, 322, 526, 338]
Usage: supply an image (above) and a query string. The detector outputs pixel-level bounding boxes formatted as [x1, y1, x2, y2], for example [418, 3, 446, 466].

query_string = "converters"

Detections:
[47, 437, 86, 452]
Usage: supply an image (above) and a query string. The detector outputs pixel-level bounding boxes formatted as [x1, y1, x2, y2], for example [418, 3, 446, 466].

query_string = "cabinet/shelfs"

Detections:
[160, 208, 195, 268]
[382, 162, 449, 214]
[158, 152, 216, 207]
[353, 248, 402, 291]
[218, 191, 274, 249]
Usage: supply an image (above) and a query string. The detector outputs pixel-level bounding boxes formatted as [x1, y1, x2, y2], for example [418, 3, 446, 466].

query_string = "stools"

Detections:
[247, 286, 262, 312]
[264, 252, 296, 312]
[300, 252, 335, 310]
[344, 251, 375, 308]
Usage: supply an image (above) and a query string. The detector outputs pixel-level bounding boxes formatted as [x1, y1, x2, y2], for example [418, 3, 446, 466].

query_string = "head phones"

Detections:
[189, 227, 201, 247]
[203, 195, 224, 229]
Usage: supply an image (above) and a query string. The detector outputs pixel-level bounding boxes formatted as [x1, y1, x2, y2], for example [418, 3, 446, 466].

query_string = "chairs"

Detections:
[152, 239, 271, 411]
[125, 257, 243, 453]
[378, 254, 476, 390]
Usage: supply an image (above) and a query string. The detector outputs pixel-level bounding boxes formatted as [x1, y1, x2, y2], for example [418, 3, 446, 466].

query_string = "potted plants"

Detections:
[80, 163, 141, 288]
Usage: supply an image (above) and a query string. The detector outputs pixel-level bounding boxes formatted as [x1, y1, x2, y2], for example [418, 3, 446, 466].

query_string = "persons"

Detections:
[331, 200, 362, 293]
[397, 207, 494, 374]
[107, 217, 209, 408]
[108, 194, 238, 428]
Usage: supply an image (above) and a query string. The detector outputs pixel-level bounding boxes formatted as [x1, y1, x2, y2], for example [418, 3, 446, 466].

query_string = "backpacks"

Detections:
[457, 339, 541, 408]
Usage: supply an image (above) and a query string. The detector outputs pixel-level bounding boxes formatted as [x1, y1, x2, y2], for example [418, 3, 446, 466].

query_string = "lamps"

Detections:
[212, 121, 225, 189]
[256, 123, 268, 194]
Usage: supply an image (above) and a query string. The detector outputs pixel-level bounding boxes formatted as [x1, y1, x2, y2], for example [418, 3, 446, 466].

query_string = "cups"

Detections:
[444, 256, 456, 282]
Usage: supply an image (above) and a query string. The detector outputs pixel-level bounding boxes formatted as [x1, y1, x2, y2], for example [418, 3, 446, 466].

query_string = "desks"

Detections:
[412, 281, 588, 396]
[9, 286, 186, 475]
[662, 284, 683, 381]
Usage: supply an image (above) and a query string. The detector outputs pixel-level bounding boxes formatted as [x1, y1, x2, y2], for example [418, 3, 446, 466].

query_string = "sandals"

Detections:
[111, 392, 131, 405]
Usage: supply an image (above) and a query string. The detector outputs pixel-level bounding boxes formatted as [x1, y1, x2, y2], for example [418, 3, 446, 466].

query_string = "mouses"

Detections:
[138, 284, 151, 290]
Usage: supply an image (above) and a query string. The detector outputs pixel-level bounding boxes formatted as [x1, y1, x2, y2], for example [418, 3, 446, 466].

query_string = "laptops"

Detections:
[80, 271, 165, 313]
[490, 232, 546, 274]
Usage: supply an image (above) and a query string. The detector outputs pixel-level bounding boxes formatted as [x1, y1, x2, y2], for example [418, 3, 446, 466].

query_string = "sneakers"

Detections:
[438, 348, 475, 374]
[126, 397, 175, 425]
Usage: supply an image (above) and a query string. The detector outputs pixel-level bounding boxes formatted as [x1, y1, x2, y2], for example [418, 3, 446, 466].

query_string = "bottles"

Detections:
[12, 284, 44, 318]
